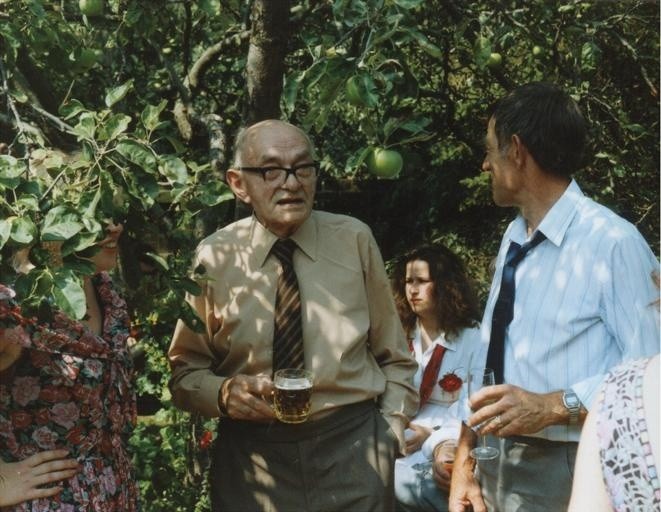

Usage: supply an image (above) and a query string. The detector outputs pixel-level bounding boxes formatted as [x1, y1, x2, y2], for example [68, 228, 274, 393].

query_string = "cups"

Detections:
[261, 367, 315, 426]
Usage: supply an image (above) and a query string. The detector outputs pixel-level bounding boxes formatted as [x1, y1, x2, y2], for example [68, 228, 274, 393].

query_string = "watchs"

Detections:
[562, 385, 584, 426]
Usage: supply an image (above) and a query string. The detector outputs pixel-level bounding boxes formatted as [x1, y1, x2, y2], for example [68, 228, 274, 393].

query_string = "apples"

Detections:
[368, 147, 402, 177]
[488, 52, 502, 67]
[346, 75, 368, 105]
[78, 0, 102, 15]
[532, 44, 543, 58]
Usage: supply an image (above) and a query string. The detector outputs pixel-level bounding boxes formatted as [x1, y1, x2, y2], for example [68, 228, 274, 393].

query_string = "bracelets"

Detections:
[219, 374, 228, 420]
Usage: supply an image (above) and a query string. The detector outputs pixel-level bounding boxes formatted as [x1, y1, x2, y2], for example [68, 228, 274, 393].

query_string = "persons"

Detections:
[448, 79, 660, 512]
[167, 119, 421, 511]
[563, 263, 661, 512]
[386, 239, 492, 512]
[0, 126, 145, 510]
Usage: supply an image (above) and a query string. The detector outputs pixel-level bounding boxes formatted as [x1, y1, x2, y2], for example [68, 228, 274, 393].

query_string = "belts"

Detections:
[504, 435, 580, 447]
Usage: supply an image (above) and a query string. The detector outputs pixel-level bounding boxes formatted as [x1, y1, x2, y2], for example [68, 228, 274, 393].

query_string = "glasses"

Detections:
[238, 160, 321, 187]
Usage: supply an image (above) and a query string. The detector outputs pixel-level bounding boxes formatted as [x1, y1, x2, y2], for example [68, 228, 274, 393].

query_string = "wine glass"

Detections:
[467, 368, 499, 462]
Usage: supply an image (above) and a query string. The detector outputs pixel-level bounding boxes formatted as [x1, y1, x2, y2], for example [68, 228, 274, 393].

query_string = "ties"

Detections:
[409, 339, 446, 409]
[476, 232, 546, 421]
[272, 239, 305, 382]
